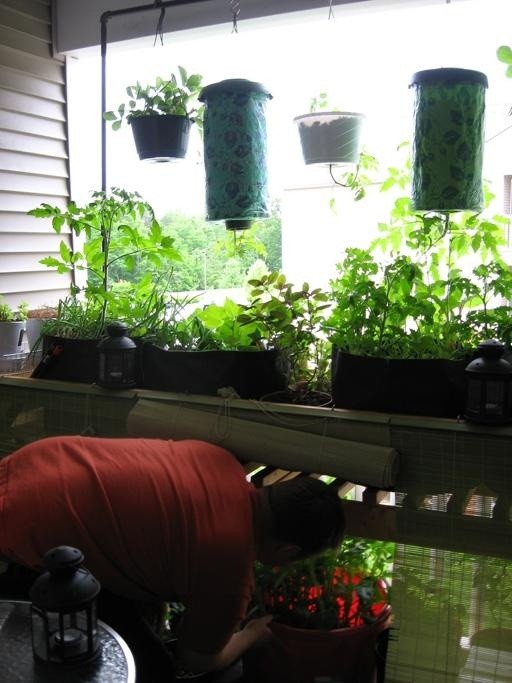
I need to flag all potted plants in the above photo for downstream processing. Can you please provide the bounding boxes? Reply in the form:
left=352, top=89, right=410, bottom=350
left=102, top=63, right=206, bottom=164
left=292, top=91, right=368, bottom=168
left=0, top=182, right=512, bottom=423
left=249, top=534, right=395, bottom=682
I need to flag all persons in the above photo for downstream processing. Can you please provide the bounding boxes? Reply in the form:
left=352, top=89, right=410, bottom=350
left=1, top=436, right=347, bottom=680
